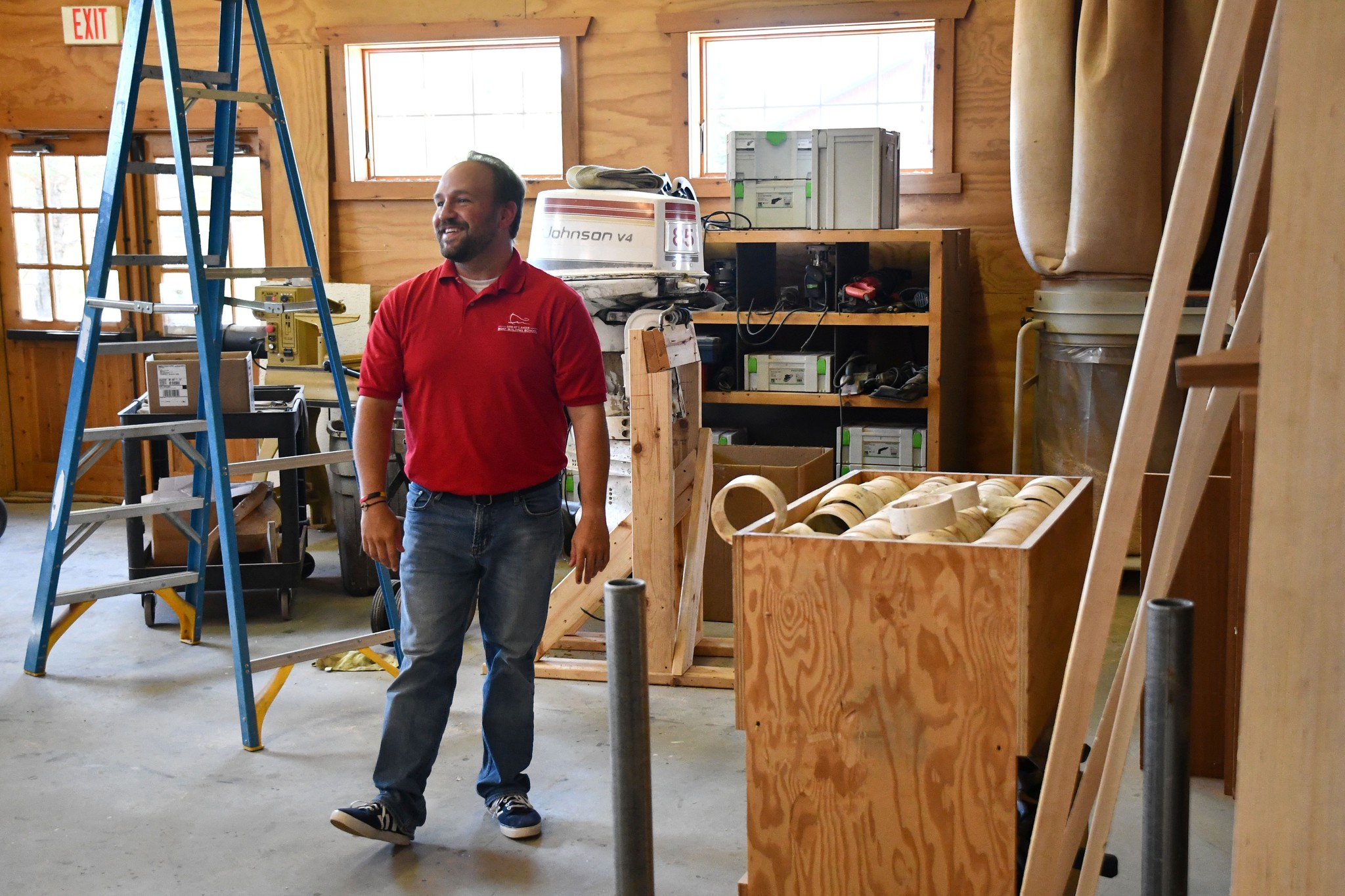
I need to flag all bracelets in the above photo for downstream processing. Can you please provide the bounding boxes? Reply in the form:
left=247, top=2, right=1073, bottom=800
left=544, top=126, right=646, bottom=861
left=359, top=492, right=388, bottom=512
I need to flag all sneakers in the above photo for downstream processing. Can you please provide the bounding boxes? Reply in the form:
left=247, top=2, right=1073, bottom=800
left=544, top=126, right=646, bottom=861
left=330, top=800, right=411, bottom=846
left=487, top=794, right=542, bottom=839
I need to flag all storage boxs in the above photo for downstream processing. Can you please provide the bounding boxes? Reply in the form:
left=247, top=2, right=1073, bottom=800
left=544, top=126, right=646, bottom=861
left=147, top=351, right=254, bottom=412
left=703, top=444, right=833, bottom=623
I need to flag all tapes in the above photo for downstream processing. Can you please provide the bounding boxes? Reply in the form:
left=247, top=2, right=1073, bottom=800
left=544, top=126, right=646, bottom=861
left=803, top=475, right=909, bottom=536
left=779, top=521, right=813, bottom=535
left=888, top=494, right=956, bottom=536
left=840, top=476, right=1074, bottom=546
left=933, top=481, right=981, bottom=511
left=709, top=473, right=787, bottom=546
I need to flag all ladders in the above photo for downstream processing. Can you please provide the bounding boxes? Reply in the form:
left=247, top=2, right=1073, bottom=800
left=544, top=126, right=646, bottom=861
left=21, top=0, right=403, bottom=752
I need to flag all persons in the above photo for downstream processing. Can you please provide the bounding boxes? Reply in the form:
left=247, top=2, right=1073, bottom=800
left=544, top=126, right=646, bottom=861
left=328, top=150, right=610, bottom=847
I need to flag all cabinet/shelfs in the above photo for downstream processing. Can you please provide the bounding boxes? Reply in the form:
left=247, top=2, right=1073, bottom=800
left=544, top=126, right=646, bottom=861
left=668, top=225, right=973, bottom=659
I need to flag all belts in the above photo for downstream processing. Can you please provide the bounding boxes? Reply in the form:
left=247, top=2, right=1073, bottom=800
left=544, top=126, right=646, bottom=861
left=437, top=473, right=559, bottom=505
